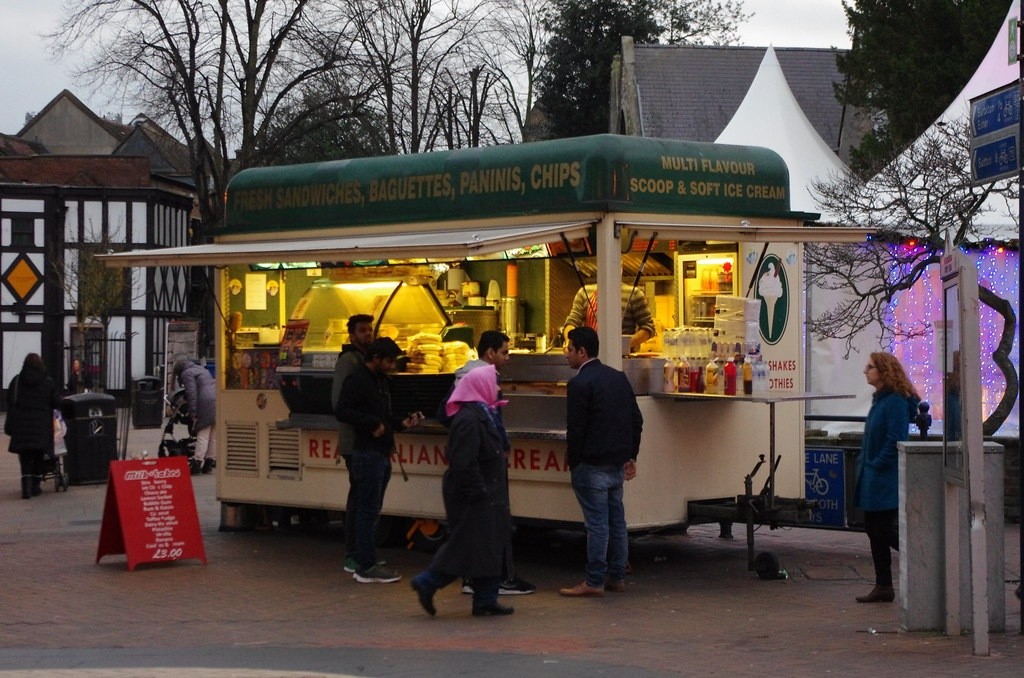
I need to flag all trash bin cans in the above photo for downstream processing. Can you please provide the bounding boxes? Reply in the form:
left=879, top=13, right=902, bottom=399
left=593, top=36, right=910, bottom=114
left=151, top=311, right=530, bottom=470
left=61, top=394, right=118, bottom=486
left=133, top=376, right=164, bottom=430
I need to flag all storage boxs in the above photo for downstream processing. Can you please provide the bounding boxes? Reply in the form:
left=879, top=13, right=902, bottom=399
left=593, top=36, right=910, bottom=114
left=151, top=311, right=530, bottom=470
left=623, top=357, right=666, bottom=394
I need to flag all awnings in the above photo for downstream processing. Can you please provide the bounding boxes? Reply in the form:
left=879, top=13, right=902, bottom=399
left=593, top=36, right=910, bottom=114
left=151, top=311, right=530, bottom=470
left=619, top=221, right=878, bottom=242
left=94, top=220, right=599, bottom=331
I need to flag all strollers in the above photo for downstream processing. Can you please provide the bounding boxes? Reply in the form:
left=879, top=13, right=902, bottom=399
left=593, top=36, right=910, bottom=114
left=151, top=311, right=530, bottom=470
left=157, top=389, right=207, bottom=474
left=41, top=407, right=73, bottom=492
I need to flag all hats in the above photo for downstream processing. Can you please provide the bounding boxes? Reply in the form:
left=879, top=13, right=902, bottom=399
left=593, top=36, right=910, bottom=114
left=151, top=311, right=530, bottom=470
left=369, top=336, right=403, bottom=358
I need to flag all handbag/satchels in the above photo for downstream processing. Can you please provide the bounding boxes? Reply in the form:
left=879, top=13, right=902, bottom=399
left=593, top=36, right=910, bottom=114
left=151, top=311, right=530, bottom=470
left=5, top=375, right=20, bottom=436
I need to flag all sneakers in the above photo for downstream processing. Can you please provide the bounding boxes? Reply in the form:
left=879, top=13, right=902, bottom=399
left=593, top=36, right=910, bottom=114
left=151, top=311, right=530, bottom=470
left=463, top=578, right=476, bottom=594
left=498, top=576, right=536, bottom=595
left=344, top=555, right=385, bottom=572
left=353, top=567, right=401, bottom=583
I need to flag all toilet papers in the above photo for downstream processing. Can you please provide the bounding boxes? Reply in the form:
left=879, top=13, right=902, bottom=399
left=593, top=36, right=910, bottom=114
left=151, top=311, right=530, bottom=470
left=448, top=268, right=466, bottom=290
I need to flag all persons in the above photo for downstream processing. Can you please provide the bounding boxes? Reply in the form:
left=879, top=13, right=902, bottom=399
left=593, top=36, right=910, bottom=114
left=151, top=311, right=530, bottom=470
left=568, top=255, right=655, bottom=350
left=856, top=352, right=919, bottom=601
left=3, top=353, right=56, bottom=500
left=172, top=359, right=216, bottom=473
left=66, top=360, right=94, bottom=394
left=557, top=327, right=644, bottom=596
left=330, top=314, right=403, bottom=584
left=409, top=330, right=537, bottom=616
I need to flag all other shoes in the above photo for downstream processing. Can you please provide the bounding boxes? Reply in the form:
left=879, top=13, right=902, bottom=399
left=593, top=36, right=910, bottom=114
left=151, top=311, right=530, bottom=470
left=855, top=590, right=894, bottom=603
left=560, top=580, right=605, bottom=596
left=472, top=603, right=514, bottom=616
left=411, top=578, right=437, bottom=615
left=606, top=579, right=625, bottom=592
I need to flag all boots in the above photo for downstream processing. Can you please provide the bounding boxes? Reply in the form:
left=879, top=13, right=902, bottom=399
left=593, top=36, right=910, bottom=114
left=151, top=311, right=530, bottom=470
left=32, top=476, right=42, bottom=496
left=21, top=475, right=33, bottom=499
left=191, top=460, right=201, bottom=474
left=202, top=459, right=213, bottom=473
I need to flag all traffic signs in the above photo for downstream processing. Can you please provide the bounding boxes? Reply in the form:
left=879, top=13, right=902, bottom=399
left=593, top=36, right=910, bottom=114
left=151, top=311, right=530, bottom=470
left=968, top=81, right=1022, bottom=138
left=966, top=134, right=1023, bottom=184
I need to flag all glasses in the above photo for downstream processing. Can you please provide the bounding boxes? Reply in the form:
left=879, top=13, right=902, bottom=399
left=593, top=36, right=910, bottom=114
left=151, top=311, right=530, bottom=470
left=868, top=364, right=877, bottom=370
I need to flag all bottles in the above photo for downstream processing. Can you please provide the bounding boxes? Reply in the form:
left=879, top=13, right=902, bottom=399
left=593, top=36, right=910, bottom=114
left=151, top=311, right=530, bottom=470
left=662, top=263, right=770, bottom=396
